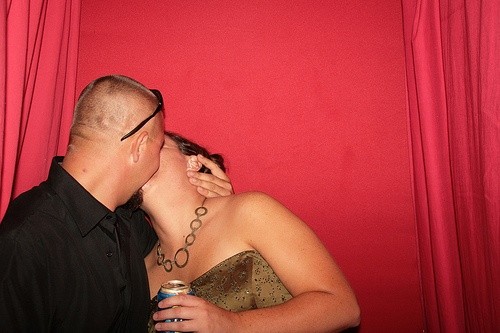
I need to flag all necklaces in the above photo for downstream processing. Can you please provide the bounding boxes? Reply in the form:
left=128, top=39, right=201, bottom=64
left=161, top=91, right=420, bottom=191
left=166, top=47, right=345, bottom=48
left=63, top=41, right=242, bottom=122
left=155, top=197, right=208, bottom=272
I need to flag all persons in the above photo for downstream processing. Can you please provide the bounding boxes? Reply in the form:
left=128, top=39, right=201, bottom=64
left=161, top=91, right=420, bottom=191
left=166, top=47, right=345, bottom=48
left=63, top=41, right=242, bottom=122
left=0, top=74, right=234, bottom=333
left=138, top=131, right=361, bottom=333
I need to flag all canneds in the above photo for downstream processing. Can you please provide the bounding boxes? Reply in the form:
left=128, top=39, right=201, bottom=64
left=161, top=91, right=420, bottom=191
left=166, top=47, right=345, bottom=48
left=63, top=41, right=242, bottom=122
left=157, top=279, right=195, bottom=333
